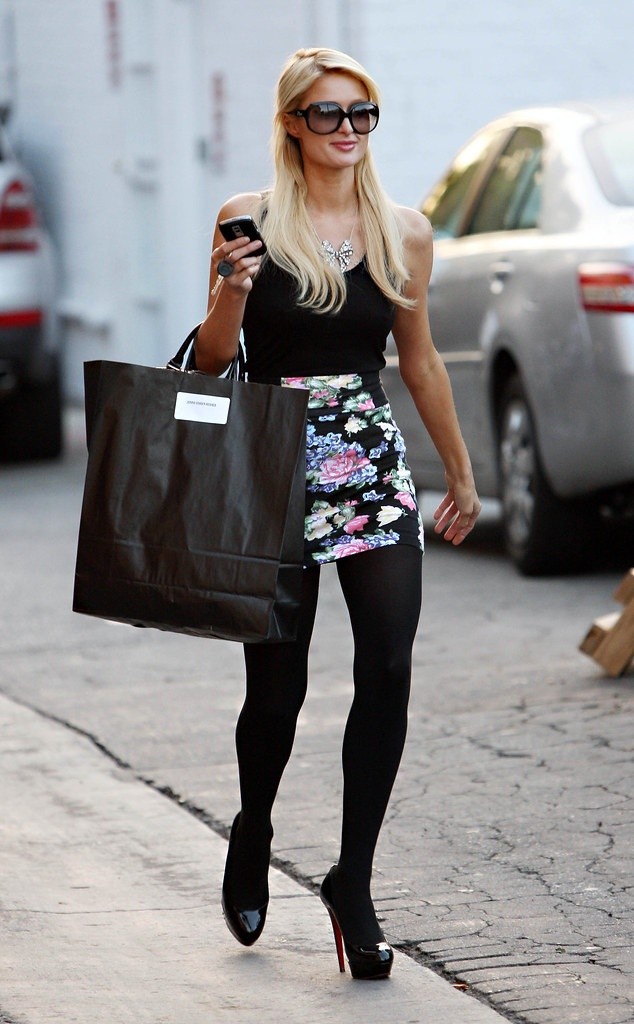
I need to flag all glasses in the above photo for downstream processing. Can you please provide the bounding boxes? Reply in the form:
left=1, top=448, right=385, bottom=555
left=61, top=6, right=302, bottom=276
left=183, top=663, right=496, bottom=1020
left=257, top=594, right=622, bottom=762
left=288, top=100, right=380, bottom=135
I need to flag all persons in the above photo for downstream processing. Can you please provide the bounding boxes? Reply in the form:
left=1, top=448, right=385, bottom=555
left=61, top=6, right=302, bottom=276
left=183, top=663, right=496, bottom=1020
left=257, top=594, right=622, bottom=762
left=189, top=49, right=480, bottom=981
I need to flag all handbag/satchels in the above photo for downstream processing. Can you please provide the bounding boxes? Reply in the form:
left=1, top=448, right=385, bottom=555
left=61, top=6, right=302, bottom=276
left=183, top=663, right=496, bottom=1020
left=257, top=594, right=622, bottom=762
left=73, top=323, right=313, bottom=644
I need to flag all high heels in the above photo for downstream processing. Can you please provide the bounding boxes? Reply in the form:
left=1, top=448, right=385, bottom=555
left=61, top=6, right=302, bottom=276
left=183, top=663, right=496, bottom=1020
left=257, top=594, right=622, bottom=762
left=319, top=865, right=394, bottom=981
left=222, top=810, right=274, bottom=947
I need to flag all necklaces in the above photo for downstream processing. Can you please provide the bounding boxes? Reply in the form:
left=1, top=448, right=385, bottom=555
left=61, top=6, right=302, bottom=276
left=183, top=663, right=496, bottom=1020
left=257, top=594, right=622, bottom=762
left=311, top=204, right=358, bottom=275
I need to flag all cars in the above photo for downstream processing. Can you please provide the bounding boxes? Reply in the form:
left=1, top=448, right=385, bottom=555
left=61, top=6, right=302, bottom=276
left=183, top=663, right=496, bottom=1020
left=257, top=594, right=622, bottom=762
left=0, top=102, right=67, bottom=466
left=382, top=103, right=634, bottom=578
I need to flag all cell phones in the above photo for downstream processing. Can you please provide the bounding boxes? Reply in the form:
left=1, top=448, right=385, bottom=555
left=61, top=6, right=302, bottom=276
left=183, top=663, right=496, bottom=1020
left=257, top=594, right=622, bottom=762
left=218, top=214, right=267, bottom=258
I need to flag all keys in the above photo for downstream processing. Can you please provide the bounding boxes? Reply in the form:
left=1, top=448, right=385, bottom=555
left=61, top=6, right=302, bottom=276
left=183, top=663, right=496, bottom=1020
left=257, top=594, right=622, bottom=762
left=210, top=252, right=235, bottom=296
